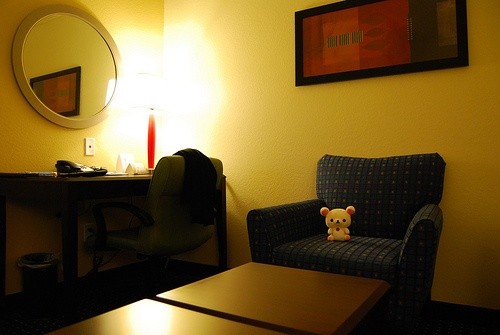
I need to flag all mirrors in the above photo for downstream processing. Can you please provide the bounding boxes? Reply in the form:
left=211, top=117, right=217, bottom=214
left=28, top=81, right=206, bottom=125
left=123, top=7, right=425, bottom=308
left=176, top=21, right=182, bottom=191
left=10, top=5, right=120, bottom=129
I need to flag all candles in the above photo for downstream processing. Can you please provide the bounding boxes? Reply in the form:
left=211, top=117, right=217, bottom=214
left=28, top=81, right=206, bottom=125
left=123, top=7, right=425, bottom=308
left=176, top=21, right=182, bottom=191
left=148, top=107, right=155, bottom=169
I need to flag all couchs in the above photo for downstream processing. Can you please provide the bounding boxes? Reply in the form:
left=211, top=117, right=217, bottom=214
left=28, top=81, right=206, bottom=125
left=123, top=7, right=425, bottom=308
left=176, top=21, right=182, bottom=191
left=247, top=151, right=447, bottom=321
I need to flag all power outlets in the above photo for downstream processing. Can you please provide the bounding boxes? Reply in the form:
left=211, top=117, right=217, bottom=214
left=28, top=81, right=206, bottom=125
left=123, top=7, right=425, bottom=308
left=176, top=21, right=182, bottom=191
left=84, top=223, right=96, bottom=242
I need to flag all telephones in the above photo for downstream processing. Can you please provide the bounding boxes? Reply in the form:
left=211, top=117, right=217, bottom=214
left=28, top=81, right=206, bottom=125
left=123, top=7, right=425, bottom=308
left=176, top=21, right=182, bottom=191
left=56, top=161, right=107, bottom=175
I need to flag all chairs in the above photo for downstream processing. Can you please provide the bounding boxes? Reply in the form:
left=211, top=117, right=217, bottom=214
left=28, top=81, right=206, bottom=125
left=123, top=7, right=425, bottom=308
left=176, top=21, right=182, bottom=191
left=84, top=154, right=223, bottom=301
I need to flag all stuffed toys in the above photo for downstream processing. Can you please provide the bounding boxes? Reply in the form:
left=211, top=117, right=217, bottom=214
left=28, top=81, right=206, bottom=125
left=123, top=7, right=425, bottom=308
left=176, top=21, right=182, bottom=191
left=319, top=206, right=355, bottom=243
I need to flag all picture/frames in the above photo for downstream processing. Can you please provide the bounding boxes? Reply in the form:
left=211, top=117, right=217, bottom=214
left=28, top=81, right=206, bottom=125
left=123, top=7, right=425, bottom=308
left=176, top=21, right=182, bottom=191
left=29, top=67, right=80, bottom=117
left=294, top=0, right=469, bottom=86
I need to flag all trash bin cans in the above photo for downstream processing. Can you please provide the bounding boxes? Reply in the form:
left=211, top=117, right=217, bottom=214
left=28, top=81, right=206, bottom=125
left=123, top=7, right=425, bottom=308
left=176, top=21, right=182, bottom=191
left=20, top=253, right=61, bottom=309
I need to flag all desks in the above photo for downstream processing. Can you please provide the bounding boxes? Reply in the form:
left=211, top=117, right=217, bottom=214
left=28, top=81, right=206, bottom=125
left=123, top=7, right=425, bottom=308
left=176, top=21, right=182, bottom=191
left=1, top=172, right=225, bottom=315
